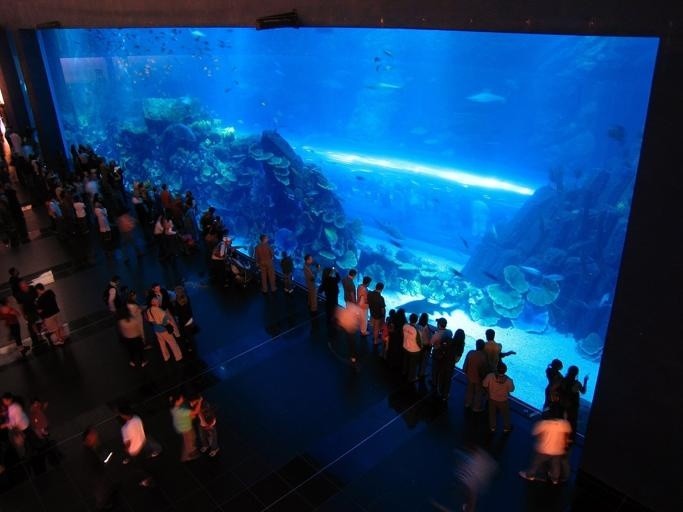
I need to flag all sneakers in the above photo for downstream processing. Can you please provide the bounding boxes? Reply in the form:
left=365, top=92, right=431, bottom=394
left=182, top=446, right=220, bottom=460
left=261, top=287, right=294, bottom=294
left=123, top=450, right=160, bottom=487
left=548, top=472, right=559, bottom=484
left=21, top=338, right=65, bottom=355
left=464, top=400, right=513, bottom=431
left=520, top=472, right=536, bottom=481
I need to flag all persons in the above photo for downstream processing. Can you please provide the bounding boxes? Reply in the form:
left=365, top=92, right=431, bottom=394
left=255, top=235, right=293, bottom=293
left=104, top=277, right=198, bottom=367
left=1, top=267, right=65, bottom=353
left=2, top=129, right=128, bottom=265
left=169, top=393, right=219, bottom=462
left=517, top=358, right=589, bottom=485
left=303, top=255, right=465, bottom=401
left=2, top=394, right=56, bottom=461
left=463, top=329, right=514, bottom=433
left=201, top=209, right=242, bottom=285
left=83, top=409, right=154, bottom=512
left=132, top=183, right=198, bottom=259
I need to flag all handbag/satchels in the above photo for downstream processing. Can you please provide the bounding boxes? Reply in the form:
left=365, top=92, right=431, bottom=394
left=8, top=423, right=26, bottom=448
